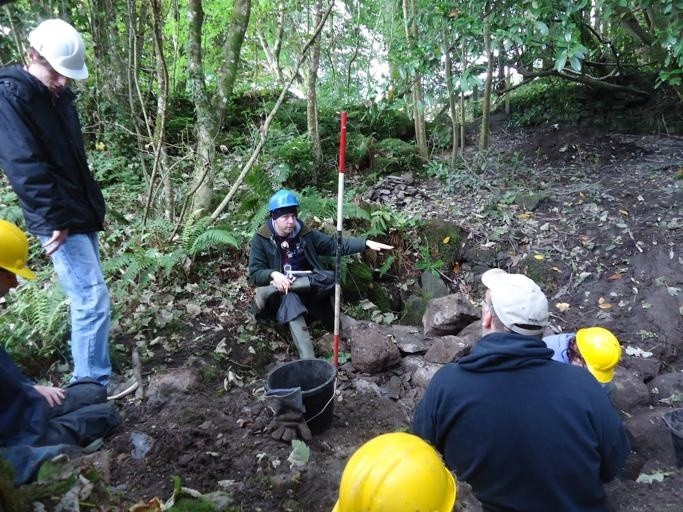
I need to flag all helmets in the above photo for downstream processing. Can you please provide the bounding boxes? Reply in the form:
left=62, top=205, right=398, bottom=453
left=28, top=18, right=90, bottom=79
left=1, top=221, right=37, bottom=281
left=270, top=190, right=301, bottom=211
left=576, top=327, right=621, bottom=384
left=330, top=433, right=455, bottom=512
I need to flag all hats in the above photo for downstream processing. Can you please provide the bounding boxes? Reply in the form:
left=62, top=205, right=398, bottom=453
left=482, top=268, right=549, bottom=335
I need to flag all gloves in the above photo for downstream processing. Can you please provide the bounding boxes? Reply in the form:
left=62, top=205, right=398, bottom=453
left=264, top=388, right=312, bottom=441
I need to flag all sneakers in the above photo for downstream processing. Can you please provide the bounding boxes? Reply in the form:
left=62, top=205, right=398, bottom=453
left=105, top=373, right=138, bottom=401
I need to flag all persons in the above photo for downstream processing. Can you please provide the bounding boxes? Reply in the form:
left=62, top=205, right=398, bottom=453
left=331, top=431, right=483, bottom=512
left=0, top=219, right=124, bottom=489
left=249, top=189, right=395, bottom=359
left=542, top=327, right=621, bottom=404
left=0, top=18, right=138, bottom=400
left=408, top=268, right=631, bottom=512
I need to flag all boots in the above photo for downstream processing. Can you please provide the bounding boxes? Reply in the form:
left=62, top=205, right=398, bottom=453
left=289, top=314, right=316, bottom=361
left=253, top=276, right=311, bottom=309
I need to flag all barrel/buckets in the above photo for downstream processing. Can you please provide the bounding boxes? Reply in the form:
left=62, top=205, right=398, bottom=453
left=263, top=359, right=338, bottom=433
